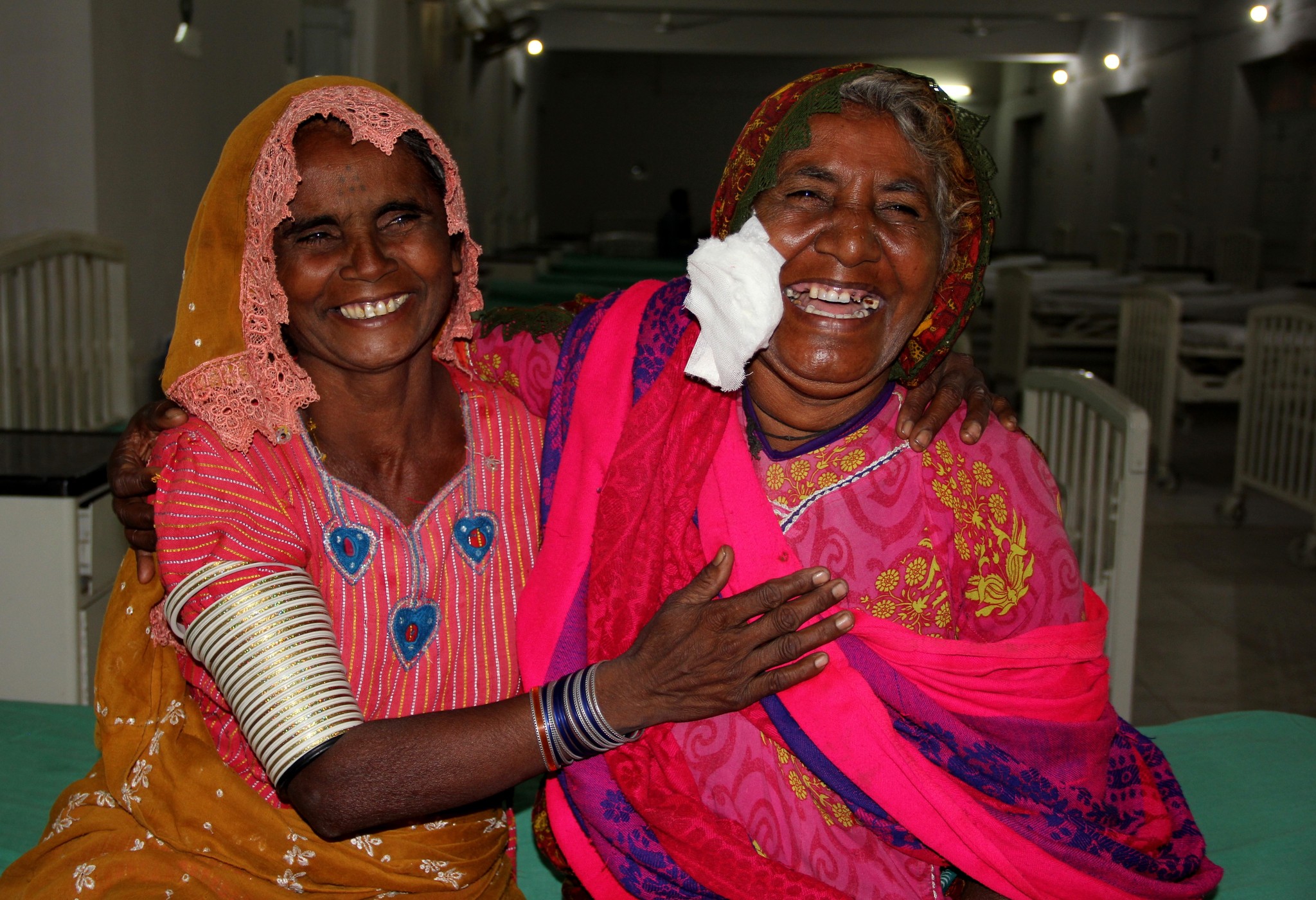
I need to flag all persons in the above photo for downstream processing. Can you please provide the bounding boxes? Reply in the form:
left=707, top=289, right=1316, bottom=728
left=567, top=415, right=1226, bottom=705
left=107, top=68, right=1229, bottom=900
left=3, top=76, right=856, bottom=900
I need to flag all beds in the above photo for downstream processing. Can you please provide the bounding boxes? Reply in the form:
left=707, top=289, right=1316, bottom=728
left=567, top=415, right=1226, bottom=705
left=1, top=692, right=1315, bottom=900
left=466, top=229, right=694, bottom=309
left=963, top=220, right=1316, bottom=555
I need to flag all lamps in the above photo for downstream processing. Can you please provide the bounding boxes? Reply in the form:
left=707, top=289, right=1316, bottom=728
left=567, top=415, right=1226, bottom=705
left=1102, top=50, right=1130, bottom=71
left=928, top=82, right=984, bottom=97
left=1251, top=0, right=1284, bottom=25
left=1052, top=69, right=1076, bottom=85
left=517, top=36, right=544, bottom=58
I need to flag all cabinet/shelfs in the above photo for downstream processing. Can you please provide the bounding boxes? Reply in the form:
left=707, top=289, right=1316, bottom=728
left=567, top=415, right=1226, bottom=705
left=0, top=426, right=130, bottom=708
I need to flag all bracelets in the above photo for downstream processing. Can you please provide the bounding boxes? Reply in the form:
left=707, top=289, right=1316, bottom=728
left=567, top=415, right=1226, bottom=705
left=529, top=660, right=650, bottom=771
